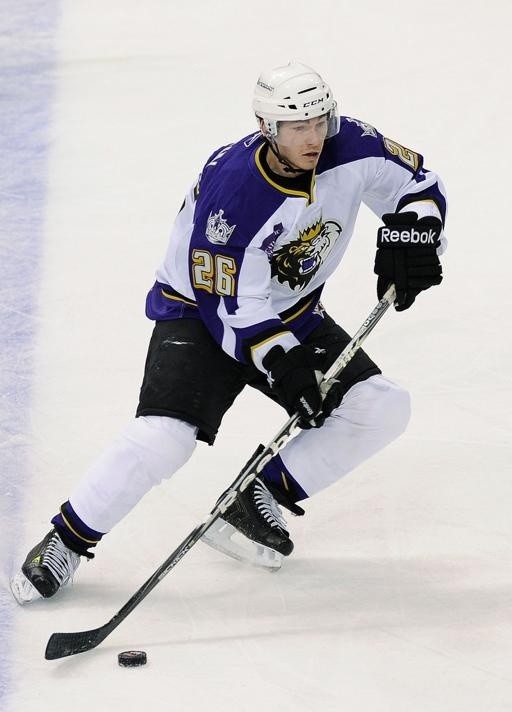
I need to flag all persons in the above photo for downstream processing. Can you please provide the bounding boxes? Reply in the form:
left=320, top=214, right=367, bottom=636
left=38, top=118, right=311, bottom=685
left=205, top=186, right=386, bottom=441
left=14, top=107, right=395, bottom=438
left=19, top=63, right=450, bottom=598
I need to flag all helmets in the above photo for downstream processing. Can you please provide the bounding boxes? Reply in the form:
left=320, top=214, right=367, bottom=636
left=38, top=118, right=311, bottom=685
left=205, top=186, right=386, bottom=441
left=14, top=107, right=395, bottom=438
left=253, top=57, right=341, bottom=147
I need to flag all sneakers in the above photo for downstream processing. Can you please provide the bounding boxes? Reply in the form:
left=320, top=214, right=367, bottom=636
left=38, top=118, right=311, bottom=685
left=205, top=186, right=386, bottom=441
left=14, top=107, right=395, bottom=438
left=23, top=522, right=94, bottom=598
left=215, top=444, right=305, bottom=557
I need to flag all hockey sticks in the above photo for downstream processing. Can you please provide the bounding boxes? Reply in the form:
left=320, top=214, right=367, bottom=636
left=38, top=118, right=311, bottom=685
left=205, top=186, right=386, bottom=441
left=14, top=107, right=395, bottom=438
left=45, top=233, right=448, bottom=660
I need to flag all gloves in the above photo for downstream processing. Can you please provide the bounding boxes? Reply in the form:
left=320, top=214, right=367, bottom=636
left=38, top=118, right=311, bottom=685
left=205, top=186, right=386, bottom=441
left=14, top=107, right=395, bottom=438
left=263, top=343, right=345, bottom=431
left=373, top=210, right=444, bottom=312
